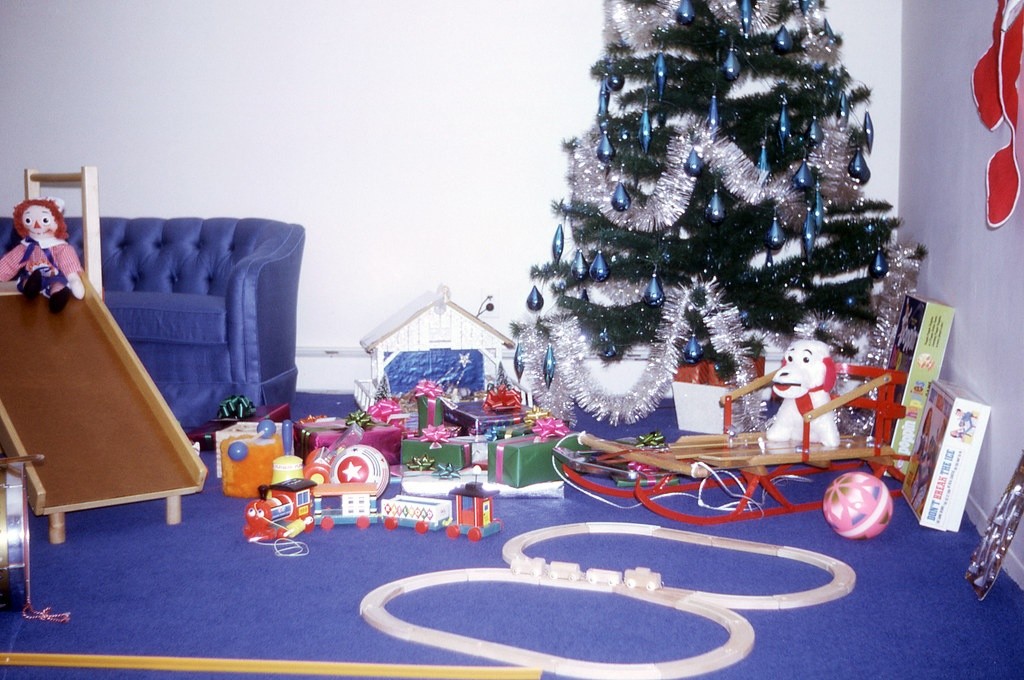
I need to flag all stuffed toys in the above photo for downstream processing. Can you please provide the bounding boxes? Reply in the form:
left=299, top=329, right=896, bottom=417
left=766, top=340, right=841, bottom=448
left=0, top=197, right=86, bottom=313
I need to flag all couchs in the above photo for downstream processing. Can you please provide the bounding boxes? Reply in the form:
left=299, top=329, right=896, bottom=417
left=0, top=217, right=305, bottom=432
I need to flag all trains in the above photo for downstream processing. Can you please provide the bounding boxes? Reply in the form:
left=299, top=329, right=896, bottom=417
left=244, top=474, right=505, bottom=546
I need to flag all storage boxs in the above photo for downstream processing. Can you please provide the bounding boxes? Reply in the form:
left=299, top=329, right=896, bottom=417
left=399, top=425, right=472, bottom=471
left=443, top=384, right=531, bottom=436
left=186, top=394, right=291, bottom=451
left=488, top=415, right=590, bottom=488
left=870, top=292, right=955, bottom=478
left=400, top=462, right=565, bottom=499
left=294, top=408, right=401, bottom=466
left=414, top=379, right=443, bottom=437
left=900, top=376, right=991, bottom=533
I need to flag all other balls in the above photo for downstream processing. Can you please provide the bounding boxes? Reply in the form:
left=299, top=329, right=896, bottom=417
left=822, top=471, right=894, bottom=540
